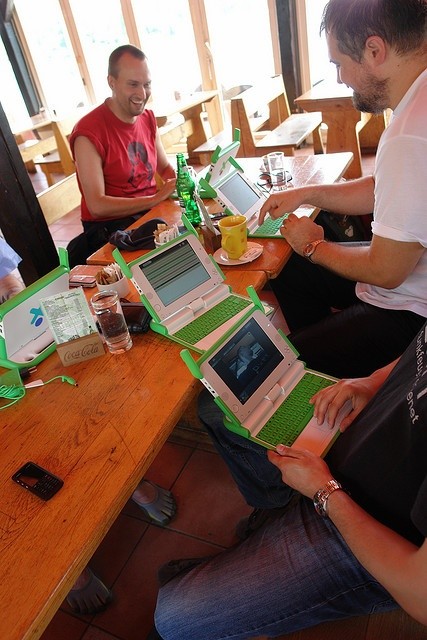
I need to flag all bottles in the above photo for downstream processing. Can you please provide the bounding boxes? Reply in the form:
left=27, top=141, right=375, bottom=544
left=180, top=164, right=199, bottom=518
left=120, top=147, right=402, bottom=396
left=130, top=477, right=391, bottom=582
left=175, top=153, right=201, bottom=229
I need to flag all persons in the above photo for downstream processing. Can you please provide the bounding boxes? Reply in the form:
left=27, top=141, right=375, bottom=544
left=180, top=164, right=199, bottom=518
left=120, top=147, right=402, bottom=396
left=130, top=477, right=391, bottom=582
left=155, top=301, right=426, bottom=635
left=1, top=228, right=178, bottom=617
left=67, top=46, right=177, bottom=248
left=260, top=1, right=426, bottom=371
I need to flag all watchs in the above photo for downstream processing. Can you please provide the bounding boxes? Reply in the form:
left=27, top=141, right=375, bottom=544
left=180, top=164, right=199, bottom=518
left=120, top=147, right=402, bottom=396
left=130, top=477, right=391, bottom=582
left=311, top=480, right=341, bottom=519
left=301, top=237, right=325, bottom=264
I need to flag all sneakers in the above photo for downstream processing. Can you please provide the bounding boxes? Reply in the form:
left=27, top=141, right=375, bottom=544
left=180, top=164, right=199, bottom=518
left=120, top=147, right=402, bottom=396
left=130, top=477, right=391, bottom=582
left=237, top=503, right=281, bottom=537
left=158, top=555, right=213, bottom=584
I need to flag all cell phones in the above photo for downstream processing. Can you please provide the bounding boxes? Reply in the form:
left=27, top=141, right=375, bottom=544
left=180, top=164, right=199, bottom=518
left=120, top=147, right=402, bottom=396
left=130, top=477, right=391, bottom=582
left=70, top=274, right=98, bottom=289
left=12, top=461, right=68, bottom=501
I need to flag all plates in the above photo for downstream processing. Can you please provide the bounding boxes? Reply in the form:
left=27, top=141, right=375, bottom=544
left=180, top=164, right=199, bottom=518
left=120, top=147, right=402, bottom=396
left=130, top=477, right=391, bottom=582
left=212, top=241, right=263, bottom=266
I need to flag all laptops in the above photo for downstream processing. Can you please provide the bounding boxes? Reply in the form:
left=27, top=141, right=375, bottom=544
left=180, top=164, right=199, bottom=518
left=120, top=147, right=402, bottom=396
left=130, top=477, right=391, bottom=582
left=1, top=247, right=71, bottom=371
left=199, top=156, right=316, bottom=240
left=111, top=213, right=275, bottom=356
left=180, top=285, right=353, bottom=460
left=171, top=128, right=239, bottom=200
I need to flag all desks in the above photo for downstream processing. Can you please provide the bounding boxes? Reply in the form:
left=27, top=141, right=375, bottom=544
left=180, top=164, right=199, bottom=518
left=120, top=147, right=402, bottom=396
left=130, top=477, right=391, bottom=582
left=85, top=152, right=354, bottom=279
left=0, top=263, right=267, bottom=640
left=151, top=91, right=220, bottom=155
left=294, top=73, right=386, bottom=178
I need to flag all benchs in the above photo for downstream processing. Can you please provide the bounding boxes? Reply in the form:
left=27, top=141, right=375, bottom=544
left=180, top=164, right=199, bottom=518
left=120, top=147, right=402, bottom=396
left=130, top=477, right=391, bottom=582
left=239, top=72, right=323, bottom=156
left=193, top=74, right=278, bottom=164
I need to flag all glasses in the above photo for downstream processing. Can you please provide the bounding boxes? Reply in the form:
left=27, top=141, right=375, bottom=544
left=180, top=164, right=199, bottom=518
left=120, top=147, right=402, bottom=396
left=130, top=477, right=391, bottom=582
left=256, top=168, right=292, bottom=197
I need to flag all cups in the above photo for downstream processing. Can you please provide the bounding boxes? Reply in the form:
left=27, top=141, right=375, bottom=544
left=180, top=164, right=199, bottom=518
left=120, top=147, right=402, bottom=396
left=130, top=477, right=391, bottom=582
left=153, top=232, right=181, bottom=249
left=260, top=155, right=275, bottom=172
left=267, top=151, right=287, bottom=193
left=91, top=290, right=134, bottom=355
left=95, top=273, right=130, bottom=298
left=217, top=214, right=248, bottom=261
left=174, top=165, right=197, bottom=179
